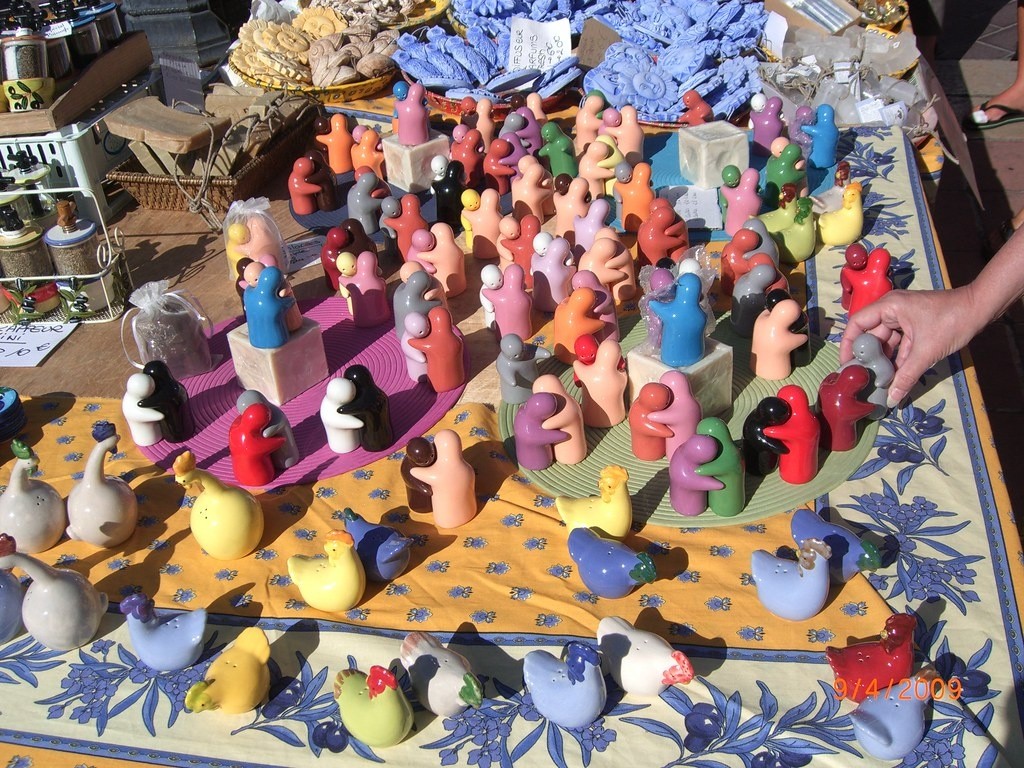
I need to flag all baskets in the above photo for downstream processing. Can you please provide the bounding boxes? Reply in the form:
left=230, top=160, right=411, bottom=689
left=105, top=109, right=317, bottom=210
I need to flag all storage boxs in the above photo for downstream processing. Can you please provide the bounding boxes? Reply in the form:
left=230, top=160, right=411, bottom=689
left=0, top=28, right=170, bottom=226
left=764, top=0, right=862, bottom=37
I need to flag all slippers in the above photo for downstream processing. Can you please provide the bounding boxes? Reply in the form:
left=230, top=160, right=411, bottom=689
left=964, top=100, right=1024, bottom=129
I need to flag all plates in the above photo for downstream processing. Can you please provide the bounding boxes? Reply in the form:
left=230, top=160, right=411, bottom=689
left=581, top=55, right=752, bottom=128
left=401, top=38, right=574, bottom=121
left=229, top=46, right=397, bottom=103
left=298, top=2, right=455, bottom=29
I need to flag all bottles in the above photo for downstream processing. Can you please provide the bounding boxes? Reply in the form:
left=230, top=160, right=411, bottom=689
left=1, top=2, right=123, bottom=103
left=0, top=163, right=115, bottom=314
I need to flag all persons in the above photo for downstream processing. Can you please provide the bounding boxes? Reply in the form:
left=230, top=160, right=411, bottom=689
left=961, top=0, right=1024, bottom=129
left=838, top=208, right=1024, bottom=408
